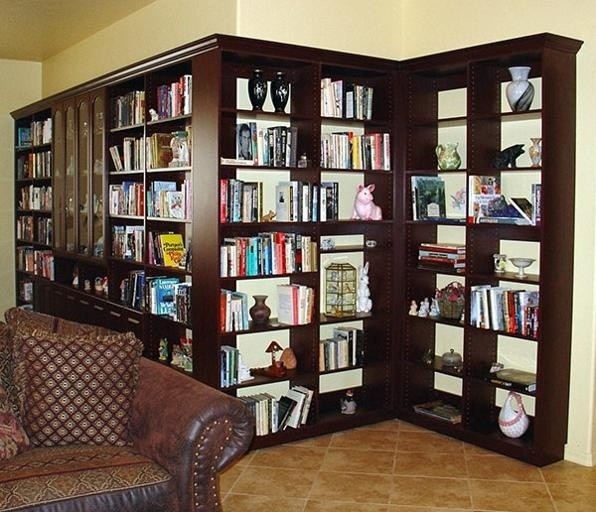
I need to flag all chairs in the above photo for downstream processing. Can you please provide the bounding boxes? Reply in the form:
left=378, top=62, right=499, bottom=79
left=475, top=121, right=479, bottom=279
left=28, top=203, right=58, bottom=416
left=1, top=316, right=258, bottom=512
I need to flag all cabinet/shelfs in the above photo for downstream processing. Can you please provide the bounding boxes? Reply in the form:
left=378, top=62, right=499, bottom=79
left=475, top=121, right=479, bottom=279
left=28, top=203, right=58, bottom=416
left=9, top=32, right=586, bottom=468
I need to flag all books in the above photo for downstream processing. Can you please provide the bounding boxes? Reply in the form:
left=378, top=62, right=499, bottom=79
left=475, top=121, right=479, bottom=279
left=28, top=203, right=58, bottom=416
left=487, top=369, right=536, bottom=393
left=220, top=345, right=314, bottom=437
left=276, top=283, right=314, bottom=326
left=220, top=288, right=250, bottom=334
left=15, top=118, right=55, bottom=282
left=410, top=174, right=541, bottom=341
left=219, top=122, right=298, bottom=167
left=274, top=181, right=339, bottom=222
left=320, top=129, right=391, bottom=171
left=321, top=76, right=374, bottom=120
left=219, top=178, right=263, bottom=223
left=319, top=326, right=364, bottom=372
left=219, top=231, right=318, bottom=278
left=109, top=72, right=192, bottom=325
